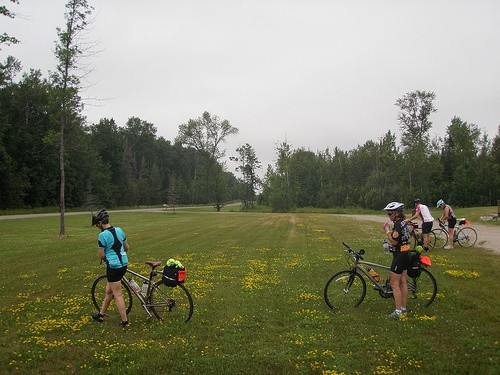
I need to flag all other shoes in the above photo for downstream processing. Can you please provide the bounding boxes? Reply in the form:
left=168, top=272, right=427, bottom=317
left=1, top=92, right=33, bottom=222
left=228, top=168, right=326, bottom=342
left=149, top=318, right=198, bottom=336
left=424, top=248, right=429, bottom=252
left=389, top=309, right=407, bottom=318
left=444, top=245, right=453, bottom=250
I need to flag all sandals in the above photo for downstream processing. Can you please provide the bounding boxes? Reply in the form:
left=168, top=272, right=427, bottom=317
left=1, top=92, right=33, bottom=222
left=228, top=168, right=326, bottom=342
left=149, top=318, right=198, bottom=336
left=119, top=321, right=129, bottom=328
left=92, top=313, right=104, bottom=323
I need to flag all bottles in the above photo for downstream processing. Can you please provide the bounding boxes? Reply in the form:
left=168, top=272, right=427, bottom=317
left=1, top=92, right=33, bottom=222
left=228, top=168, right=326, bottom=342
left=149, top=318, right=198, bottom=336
left=367, top=267, right=382, bottom=283
left=385, top=224, right=389, bottom=233
left=141, top=280, right=149, bottom=297
left=382, top=239, right=390, bottom=255
left=128, top=278, right=141, bottom=293
left=415, top=230, right=418, bottom=238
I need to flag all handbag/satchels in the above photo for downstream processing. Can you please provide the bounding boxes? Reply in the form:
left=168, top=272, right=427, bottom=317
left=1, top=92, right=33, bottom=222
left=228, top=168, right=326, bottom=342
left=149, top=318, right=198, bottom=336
left=162, top=265, right=187, bottom=288
left=407, top=250, right=420, bottom=278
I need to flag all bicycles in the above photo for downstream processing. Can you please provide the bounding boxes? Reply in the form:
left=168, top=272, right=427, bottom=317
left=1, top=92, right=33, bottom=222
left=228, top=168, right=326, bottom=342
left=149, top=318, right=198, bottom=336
left=324, top=243, right=438, bottom=311
left=409, top=221, right=436, bottom=251
left=429, top=217, right=477, bottom=248
left=92, top=259, right=193, bottom=324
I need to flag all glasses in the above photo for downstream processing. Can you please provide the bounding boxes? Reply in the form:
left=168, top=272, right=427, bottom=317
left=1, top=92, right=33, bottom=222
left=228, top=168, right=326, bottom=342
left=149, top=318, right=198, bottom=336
left=388, top=212, right=395, bottom=216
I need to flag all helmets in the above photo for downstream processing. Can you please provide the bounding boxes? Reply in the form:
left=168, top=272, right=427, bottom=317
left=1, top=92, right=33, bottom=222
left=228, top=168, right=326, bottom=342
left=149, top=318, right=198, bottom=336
left=437, top=199, right=443, bottom=209
left=92, top=209, right=109, bottom=226
left=384, top=202, right=404, bottom=211
left=413, top=199, right=421, bottom=205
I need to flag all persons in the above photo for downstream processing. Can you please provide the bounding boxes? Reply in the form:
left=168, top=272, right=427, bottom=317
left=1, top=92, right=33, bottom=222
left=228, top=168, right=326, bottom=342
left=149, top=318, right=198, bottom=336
left=406, top=199, right=434, bottom=252
left=92, top=208, right=130, bottom=327
left=436, top=199, right=457, bottom=249
left=382, top=202, right=411, bottom=319
